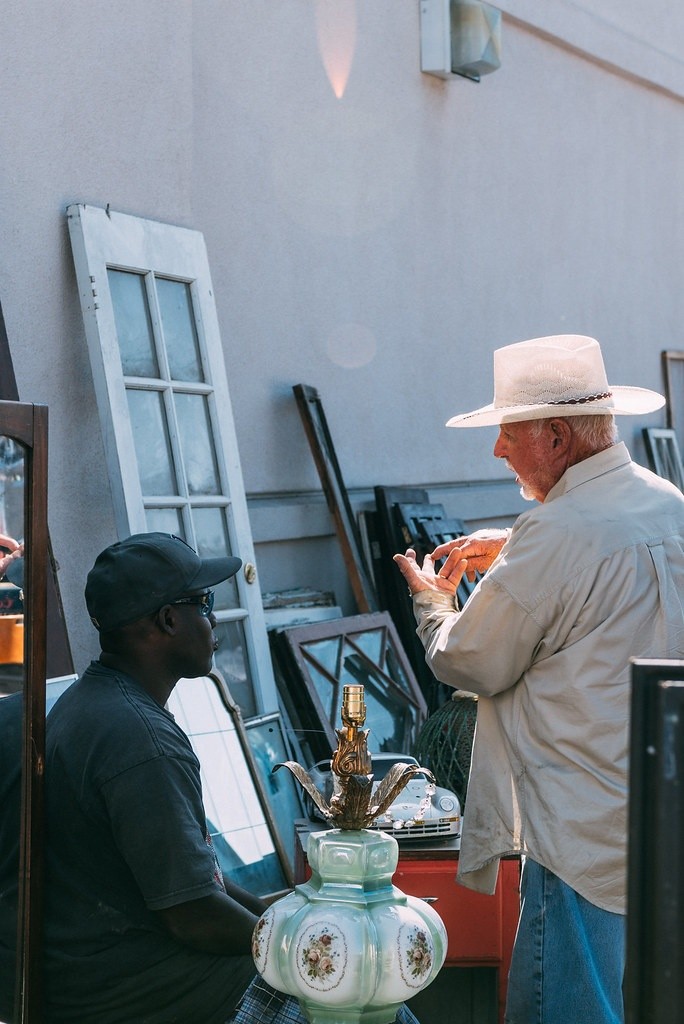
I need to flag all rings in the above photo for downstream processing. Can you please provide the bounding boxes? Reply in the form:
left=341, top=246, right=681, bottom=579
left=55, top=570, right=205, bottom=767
left=438, top=573, right=447, bottom=579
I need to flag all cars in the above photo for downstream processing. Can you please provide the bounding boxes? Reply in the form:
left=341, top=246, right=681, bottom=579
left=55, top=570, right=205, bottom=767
left=301, top=751, right=462, bottom=845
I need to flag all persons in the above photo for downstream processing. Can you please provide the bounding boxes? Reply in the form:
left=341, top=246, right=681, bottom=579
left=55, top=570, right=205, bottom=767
left=43, top=533, right=420, bottom=1024
left=393, top=334, right=684, bottom=1024
left=0, top=535, right=24, bottom=615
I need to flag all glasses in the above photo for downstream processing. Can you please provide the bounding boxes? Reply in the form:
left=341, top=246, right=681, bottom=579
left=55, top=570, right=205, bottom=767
left=169, top=591, right=215, bottom=617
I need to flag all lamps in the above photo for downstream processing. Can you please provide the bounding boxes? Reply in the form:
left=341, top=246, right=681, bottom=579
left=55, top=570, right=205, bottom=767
left=419, top=0, right=501, bottom=83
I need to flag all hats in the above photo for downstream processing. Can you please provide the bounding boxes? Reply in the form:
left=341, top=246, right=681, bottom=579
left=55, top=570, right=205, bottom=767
left=4, top=556, right=25, bottom=590
left=445, top=334, right=666, bottom=428
left=85, top=532, right=242, bottom=630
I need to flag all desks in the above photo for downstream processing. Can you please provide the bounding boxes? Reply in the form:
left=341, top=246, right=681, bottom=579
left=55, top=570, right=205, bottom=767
left=305, top=818, right=522, bottom=1024
